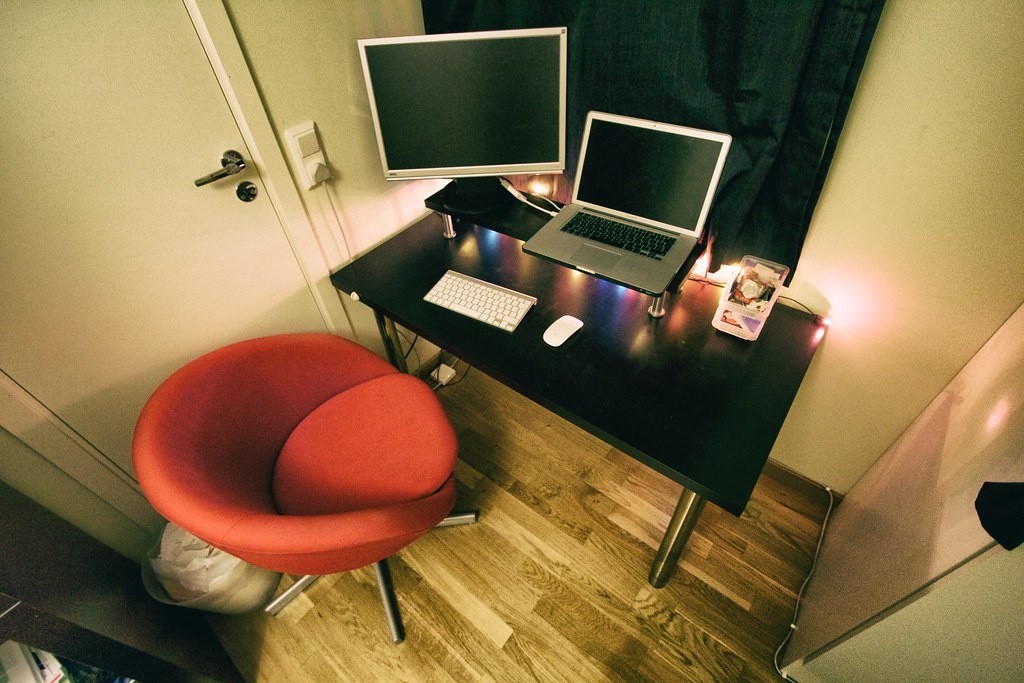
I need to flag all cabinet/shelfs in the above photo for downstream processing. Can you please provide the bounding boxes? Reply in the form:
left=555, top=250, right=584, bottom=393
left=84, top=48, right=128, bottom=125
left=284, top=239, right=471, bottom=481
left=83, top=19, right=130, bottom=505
left=780, top=302, right=1024, bottom=683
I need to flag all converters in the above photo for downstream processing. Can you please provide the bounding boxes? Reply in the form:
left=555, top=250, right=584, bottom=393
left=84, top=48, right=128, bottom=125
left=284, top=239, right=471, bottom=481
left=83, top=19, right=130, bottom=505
left=431, top=364, right=456, bottom=385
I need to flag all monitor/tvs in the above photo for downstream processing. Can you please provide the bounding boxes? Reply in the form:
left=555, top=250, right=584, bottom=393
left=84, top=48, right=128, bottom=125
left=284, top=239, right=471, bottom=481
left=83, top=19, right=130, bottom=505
left=357, top=26, right=567, bottom=213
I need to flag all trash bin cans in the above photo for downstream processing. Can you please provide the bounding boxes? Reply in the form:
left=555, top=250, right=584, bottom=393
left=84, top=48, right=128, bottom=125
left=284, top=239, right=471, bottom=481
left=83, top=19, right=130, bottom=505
left=138, top=523, right=283, bottom=622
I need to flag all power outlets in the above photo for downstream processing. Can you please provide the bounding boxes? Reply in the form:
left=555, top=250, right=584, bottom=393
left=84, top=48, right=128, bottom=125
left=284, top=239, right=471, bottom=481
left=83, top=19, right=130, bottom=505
left=283, top=119, right=335, bottom=192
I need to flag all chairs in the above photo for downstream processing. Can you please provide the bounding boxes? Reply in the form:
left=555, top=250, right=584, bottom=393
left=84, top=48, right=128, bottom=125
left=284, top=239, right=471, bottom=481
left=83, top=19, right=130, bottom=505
left=133, top=332, right=478, bottom=644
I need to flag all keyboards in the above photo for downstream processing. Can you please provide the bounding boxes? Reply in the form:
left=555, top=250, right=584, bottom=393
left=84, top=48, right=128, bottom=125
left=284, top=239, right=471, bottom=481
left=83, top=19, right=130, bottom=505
left=423, top=269, right=538, bottom=332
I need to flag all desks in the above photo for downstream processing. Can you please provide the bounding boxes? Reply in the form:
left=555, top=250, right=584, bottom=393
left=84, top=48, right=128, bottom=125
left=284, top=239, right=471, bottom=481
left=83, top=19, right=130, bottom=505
left=329, top=176, right=828, bottom=589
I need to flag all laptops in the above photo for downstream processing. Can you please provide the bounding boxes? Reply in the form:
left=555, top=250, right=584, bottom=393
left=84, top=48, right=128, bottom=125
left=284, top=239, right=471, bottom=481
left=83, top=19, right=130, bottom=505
left=522, top=111, right=732, bottom=296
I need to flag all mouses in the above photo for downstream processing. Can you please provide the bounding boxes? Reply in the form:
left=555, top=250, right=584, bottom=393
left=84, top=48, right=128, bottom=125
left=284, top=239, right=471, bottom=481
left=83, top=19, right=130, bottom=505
left=543, top=315, right=584, bottom=347
